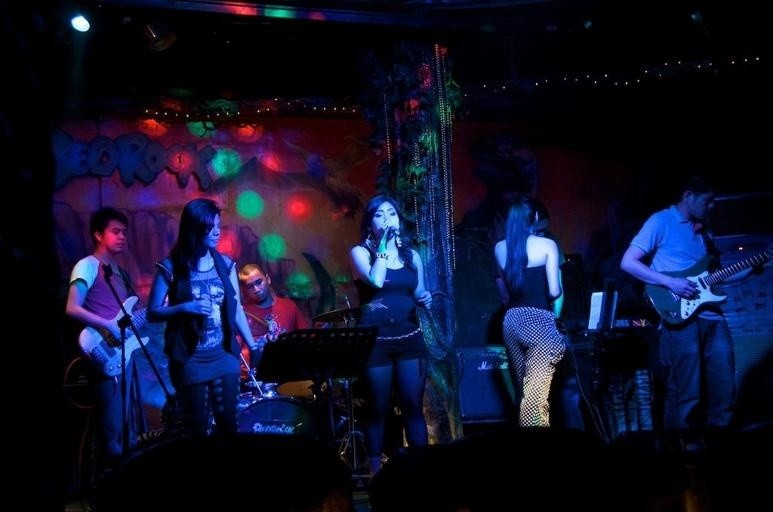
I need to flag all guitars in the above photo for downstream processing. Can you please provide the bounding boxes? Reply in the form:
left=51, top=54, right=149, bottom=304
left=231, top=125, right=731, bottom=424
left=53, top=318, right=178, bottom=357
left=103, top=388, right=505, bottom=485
left=79, top=296, right=168, bottom=377
left=644, top=247, right=773, bottom=326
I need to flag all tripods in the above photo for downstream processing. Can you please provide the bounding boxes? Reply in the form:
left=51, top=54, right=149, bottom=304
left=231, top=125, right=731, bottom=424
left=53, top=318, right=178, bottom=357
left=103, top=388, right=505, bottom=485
left=340, top=380, right=367, bottom=470
left=117, top=328, right=168, bottom=456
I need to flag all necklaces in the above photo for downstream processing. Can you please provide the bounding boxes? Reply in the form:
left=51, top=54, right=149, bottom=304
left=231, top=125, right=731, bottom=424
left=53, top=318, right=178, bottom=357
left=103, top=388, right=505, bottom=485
left=197, top=255, right=212, bottom=294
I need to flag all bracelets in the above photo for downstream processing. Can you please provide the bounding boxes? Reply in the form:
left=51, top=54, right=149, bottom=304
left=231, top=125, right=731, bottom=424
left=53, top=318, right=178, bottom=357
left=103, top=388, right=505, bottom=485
left=375, top=251, right=389, bottom=260
left=250, top=343, right=257, bottom=349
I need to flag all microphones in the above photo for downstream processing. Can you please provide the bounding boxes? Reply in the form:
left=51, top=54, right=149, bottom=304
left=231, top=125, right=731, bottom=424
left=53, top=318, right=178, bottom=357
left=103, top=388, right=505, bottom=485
left=201, top=293, right=210, bottom=342
left=388, top=226, right=400, bottom=236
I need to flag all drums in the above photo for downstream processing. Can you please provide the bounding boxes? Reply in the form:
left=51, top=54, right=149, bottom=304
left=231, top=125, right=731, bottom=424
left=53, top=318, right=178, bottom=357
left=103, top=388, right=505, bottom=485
left=235, top=388, right=306, bottom=436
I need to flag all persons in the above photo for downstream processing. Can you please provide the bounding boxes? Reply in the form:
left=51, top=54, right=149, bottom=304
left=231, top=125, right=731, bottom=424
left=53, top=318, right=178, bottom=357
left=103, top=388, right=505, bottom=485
left=145, top=198, right=258, bottom=429
left=494, top=200, right=565, bottom=426
left=621, top=177, right=738, bottom=436
left=351, top=196, right=431, bottom=476
left=233, top=263, right=309, bottom=389
left=66, top=207, right=134, bottom=461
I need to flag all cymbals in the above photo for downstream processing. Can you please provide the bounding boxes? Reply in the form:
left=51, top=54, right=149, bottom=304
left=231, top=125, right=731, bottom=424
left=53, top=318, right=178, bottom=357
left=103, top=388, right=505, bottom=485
left=312, top=306, right=361, bottom=323
left=275, top=379, right=315, bottom=399
